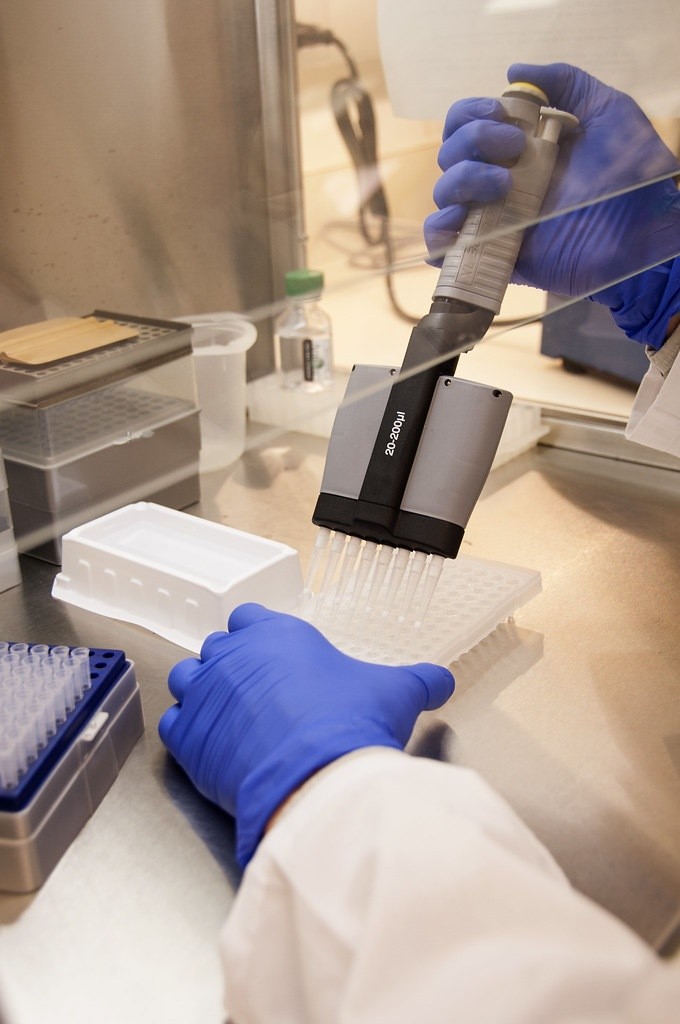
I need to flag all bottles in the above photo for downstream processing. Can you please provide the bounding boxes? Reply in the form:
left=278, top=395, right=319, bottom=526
left=277, top=270, right=333, bottom=400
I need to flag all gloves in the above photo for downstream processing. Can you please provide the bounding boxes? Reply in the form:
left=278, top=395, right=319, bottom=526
left=422, top=63, right=680, bottom=354
left=158, top=601, right=455, bottom=871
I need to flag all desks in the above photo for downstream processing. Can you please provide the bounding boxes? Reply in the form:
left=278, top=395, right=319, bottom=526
left=0, top=263, right=679, bottom=1024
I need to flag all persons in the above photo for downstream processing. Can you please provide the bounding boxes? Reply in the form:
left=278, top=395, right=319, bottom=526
left=157, top=60, right=680, bottom=1024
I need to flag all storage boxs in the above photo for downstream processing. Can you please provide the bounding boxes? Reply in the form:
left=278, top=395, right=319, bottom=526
left=0, top=310, right=201, bottom=566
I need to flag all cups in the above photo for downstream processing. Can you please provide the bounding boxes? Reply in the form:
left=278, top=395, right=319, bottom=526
left=171, top=318, right=257, bottom=473
